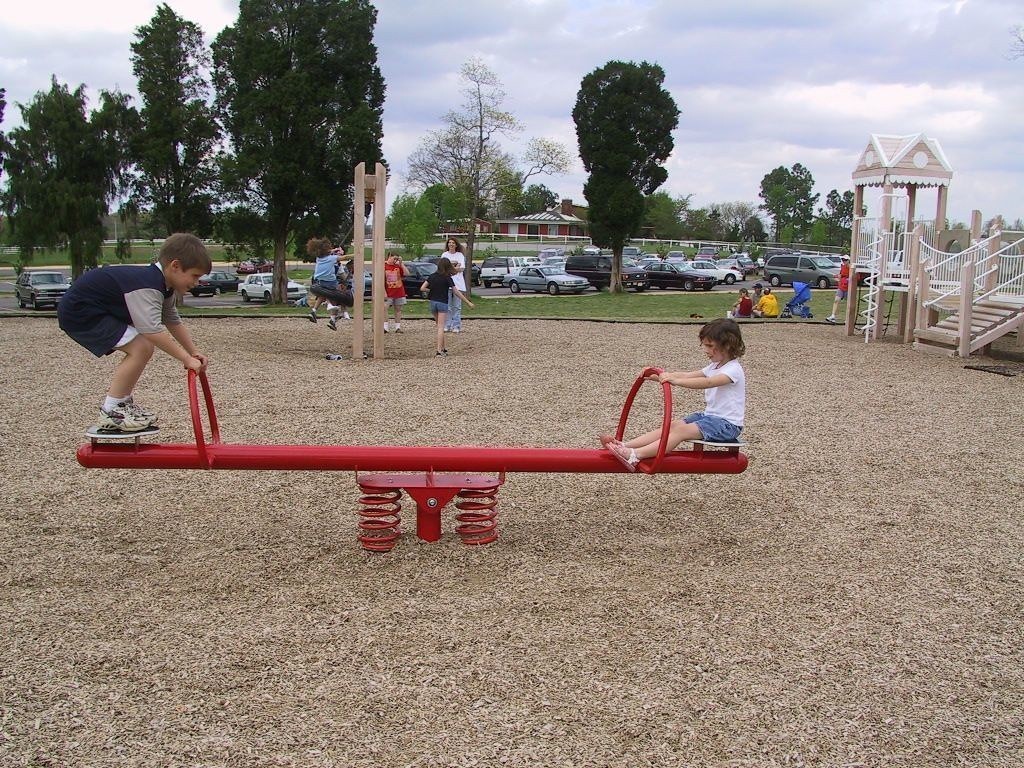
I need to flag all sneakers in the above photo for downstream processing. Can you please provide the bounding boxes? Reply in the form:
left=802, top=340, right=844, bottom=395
left=97, top=406, right=150, bottom=432
left=825, top=317, right=835, bottom=322
left=309, top=312, right=318, bottom=323
left=327, top=354, right=341, bottom=360
left=125, top=397, right=158, bottom=423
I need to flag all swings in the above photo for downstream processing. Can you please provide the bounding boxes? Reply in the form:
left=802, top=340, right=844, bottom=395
left=310, top=215, right=373, bottom=307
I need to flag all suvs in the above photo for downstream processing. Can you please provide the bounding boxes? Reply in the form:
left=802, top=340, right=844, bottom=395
left=762, top=254, right=842, bottom=289
left=14, top=271, right=72, bottom=310
left=565, top=254, right=648, bottom=292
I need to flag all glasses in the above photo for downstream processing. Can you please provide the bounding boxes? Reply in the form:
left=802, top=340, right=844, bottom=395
left=841, top=259, right=848, bottom=261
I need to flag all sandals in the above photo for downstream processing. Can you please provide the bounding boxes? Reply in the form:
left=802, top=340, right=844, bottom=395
left=606, top=442, right=640, bottom=472
left=600, top=433, right=625, bottom=447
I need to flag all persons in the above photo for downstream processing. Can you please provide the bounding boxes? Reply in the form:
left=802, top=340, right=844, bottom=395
left=57, top=233, right=213, bottom=432
left=600, top=321, right=746, bottom=472
left=384, top=252, right=409, bottom=334
left=307, top=237, right=355, bottom=331
left=826, top=254, right=860, bottom=322
left=441, top=237, right=466, bottom=333
left=731, top=283, right=779, bottom=318
left=419, top=258, right=474, bottom=355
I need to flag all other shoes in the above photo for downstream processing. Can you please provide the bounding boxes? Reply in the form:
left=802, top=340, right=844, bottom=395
left=453, top=329, right=459, bottom=333
left=437, top=351, right=448, bottom=357
left=395, top=328, right=402, bottom=333
left=327, top=319, right=337, bottom=330
left=444, top=329, right=449, bottom=331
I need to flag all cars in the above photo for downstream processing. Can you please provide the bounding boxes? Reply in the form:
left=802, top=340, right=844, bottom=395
left=189, top=271, right=245, bottom=297
left=501, top=265, right=591, bottom=296
left=235, top=257, right=274, bottom=274
left=403, top=261, right=438, bottom=299
left=347, top=270, right=373, bottom=297
left=237, top=272, right=308, bottom=304
left=421, top=244, right=933, bottom=288
left=642, top=263, right=718, bottom=292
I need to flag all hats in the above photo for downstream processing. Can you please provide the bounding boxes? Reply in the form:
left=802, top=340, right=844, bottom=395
left=752, top=284, right=762, bottom=288
left=841, top=255, right=850, bottom=259
